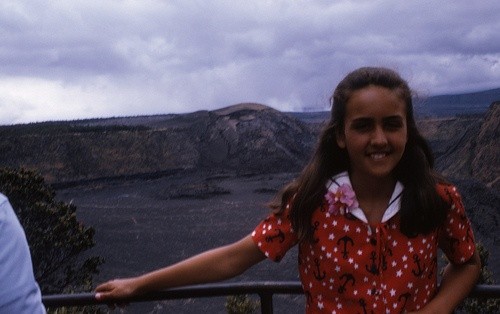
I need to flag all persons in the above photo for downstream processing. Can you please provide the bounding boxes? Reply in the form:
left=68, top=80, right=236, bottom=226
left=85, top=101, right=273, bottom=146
left=0, top=191, right=46, bottom=314
left=96, top=66, right=482, bottom=314
